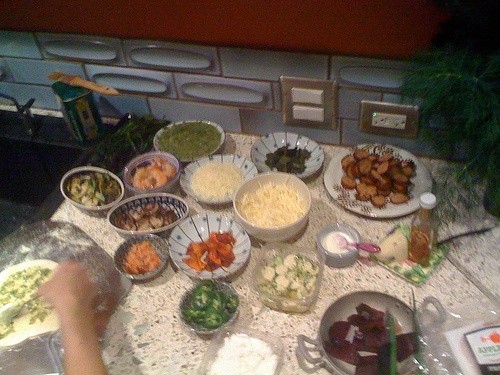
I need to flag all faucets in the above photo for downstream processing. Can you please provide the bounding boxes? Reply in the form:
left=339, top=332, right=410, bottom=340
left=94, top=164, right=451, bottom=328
left=0, top=91, right=20, bottom=110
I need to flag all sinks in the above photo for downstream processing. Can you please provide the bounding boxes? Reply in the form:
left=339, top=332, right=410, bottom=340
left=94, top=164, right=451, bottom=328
left=0, top=133, right=95, bottom=221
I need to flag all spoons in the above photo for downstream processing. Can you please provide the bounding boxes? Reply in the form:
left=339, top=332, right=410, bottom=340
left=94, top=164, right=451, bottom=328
left=334, top=235, right=381, bottom=254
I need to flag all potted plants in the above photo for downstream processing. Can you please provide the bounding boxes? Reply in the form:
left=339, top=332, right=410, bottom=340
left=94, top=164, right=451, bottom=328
left=399, top=0, right=500, bottom=236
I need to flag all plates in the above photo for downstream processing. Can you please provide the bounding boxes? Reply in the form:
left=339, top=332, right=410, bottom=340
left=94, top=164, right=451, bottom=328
left=324, top=143, right=432, bottom=218
left=250, top=131, right=324, bottom=178
left=169, top=214, right=252, bottom=280
left=179, top=280, right=239, bottom=334
left=153, top=120, right=225, bottom=161
left=180, top=154, right=258, bottom=203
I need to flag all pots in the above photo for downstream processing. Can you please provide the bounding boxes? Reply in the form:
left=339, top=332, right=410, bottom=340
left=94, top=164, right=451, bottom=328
left=297, top=291, right=446, bottom=375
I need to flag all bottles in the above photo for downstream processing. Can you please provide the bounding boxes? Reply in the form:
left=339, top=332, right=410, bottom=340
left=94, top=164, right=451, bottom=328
left=408, top=192, right=440, bottom=262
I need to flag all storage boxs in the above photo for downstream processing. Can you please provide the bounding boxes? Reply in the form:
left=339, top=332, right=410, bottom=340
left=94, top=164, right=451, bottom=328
left=52, top=81, right=106, bottom=145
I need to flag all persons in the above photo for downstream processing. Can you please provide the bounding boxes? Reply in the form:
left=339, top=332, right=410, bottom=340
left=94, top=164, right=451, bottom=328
left=36, top=262, right=110, bottom=375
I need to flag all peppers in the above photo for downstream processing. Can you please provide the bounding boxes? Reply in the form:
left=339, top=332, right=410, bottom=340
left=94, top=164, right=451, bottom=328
left=182, top=278, right=239, bottom=330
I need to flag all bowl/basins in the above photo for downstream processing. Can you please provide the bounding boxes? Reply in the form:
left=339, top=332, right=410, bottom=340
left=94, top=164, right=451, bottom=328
left=113, top=234, right=168, bottom=280
left=107, top=193, right=189, bottom=239
left=233, top=173, right=311, bottom=241
left=196, top=325, right=284, bottom=375
left=316, top=223, right=360, bottom=267
left=60, top=166, right=124, bottom=210
left=123, top=152, right=180, bottom=193
left=249, top=242, right=322, bottom=312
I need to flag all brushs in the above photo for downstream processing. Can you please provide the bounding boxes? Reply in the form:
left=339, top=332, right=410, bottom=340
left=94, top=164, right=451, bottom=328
left=48, top=70, right=122, bottom=96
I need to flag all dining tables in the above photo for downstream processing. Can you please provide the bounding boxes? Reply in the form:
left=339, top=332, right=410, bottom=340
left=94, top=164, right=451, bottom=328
left=48, top=133, right=500, bottom=375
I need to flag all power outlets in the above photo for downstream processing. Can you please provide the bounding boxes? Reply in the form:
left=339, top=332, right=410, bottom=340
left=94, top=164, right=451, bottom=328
left=359, top=101, right=420, bottom=139
